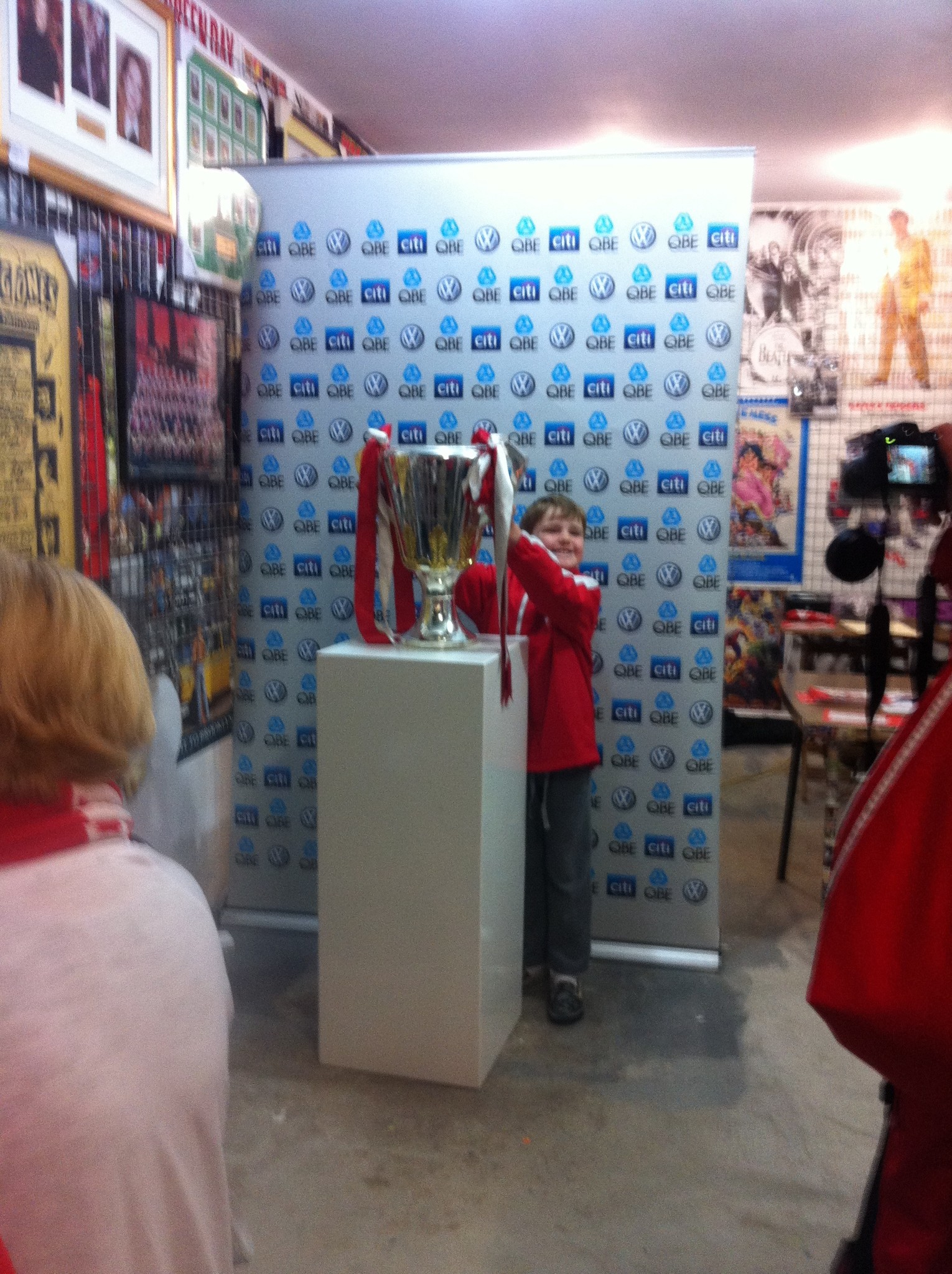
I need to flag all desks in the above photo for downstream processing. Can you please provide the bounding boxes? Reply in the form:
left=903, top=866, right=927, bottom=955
left=842, top=619, right=921, bottom=671
left=777, top=673, right=934, bottom=880
left=319, top=634, right=529, bottom=1089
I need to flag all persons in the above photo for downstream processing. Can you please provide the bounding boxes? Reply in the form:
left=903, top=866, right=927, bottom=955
left=0, top=544, right=235, bottom=1274
left=863, top=207, right=933, bottom=389
left=118, top=46, right=151, bottom=151
left=804, top=662, right=952, bottom=1274
left=128, top=362, right=224, bottom=462
left=71, top=0, right=110, bottom=107
left=20, top=0, right=60, bottom=100
left=452, top=492, right=602, bottom=1025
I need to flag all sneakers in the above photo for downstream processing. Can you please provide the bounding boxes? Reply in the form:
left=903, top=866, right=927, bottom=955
left=550, top=975, right=585, bottom=1023
left=522, top=966, right=545, bottom=995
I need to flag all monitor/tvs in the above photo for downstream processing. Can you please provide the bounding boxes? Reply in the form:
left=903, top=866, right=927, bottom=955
left=120, top=288, right=230, bottom=484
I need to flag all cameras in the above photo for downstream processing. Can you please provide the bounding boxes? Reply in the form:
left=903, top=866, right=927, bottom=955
left=840, top=421, right=950, bottom=497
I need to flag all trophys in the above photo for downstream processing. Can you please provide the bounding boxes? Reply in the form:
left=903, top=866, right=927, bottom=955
left=366, top=429, right=529, bottom=649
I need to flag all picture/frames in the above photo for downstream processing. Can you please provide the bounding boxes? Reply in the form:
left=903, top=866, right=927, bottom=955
left=123, top=288, right=228, bottom=482
